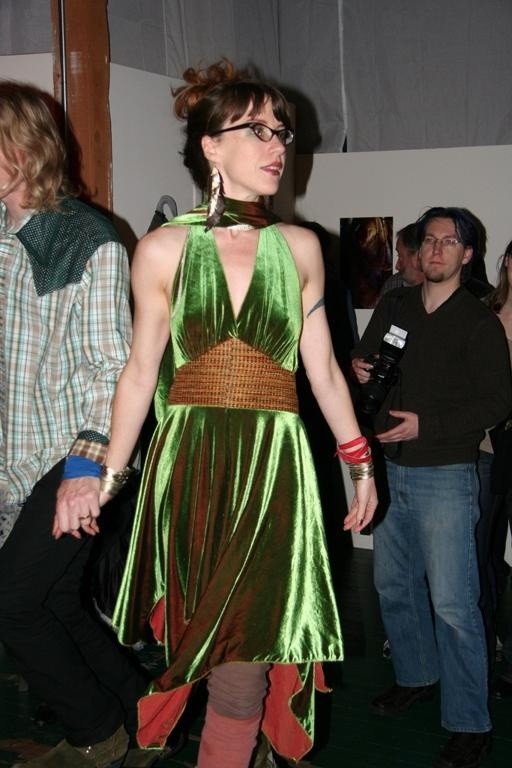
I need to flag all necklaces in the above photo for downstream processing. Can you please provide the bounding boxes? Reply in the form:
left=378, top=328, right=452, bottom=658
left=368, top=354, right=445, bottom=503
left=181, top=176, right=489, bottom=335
left=226, top=221, right=254, bottom=232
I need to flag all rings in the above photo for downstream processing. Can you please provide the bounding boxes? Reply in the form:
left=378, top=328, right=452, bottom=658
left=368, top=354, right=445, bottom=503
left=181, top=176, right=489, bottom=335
left=80, top=513, right=91, bottom=521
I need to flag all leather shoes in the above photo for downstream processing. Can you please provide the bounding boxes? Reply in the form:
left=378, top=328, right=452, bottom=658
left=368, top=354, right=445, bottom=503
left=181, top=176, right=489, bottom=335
left=430, top=729, right=494, bottom=768
left=369, top=679, right=440, bottom=717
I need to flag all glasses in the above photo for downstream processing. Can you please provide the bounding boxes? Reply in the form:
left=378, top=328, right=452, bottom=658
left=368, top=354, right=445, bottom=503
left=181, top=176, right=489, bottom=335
left=420, top=235, right=463, bottom=248
left=207, top=121, right=296, bottom=148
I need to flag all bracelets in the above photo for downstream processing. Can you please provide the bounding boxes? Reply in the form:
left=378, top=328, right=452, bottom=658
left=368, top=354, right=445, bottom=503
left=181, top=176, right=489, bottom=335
left=334, top=435, right=374, bottom=464
left=344, top=452, right=375, bottom=479
left=99, top=464, right=132, bottom=496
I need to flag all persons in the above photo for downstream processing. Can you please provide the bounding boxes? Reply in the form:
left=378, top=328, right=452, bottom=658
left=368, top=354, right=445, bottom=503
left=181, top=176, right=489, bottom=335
left=351, top=205, right=509, bottom=768
left=1, top=74, right=187, bottom=764
left=51, top=54, right=381, bottom=766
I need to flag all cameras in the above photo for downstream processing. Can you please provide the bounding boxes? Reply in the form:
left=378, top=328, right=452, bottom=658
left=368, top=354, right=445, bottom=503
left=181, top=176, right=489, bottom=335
left=359, top=333, right=406, bottom=414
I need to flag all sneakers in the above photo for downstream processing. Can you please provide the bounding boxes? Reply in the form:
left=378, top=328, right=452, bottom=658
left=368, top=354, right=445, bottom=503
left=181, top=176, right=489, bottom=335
left=10, top=722, right=175, bottom=768
left=488, top=678, right=512, bottom=704
